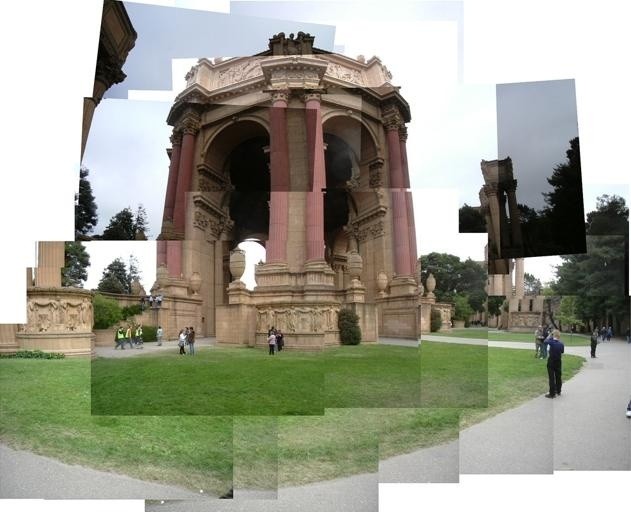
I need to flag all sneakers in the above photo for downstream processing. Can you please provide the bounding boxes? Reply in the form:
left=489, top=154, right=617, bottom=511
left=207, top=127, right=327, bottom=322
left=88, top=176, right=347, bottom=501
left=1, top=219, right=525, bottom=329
left=625, top=410, right=631, bottom=416
left=545, top=391, right=561, bottom=398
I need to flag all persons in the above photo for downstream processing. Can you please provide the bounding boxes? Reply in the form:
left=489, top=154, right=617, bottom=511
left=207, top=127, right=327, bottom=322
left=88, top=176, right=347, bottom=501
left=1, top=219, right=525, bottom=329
left=607, top=326, right=614, bottom=341
left=135, top=327, right=142, bottom=346
left=114, top=326, right=127, bottom=350
left=269, top=327, right=278, bottom=335
left=601, top=326, right=608, bottom=341
left=138, top=324, right=144, bottom=347
left=543, top=329, right=565, bottom=398
left=184, top=327, right=190, bottom=345
left=535, top=325, right=546, bottom=358
left=627, top=328, right=631, bottom=343
left=591, top=331, right=598, bottom=358
left=275, top=329, right=285, bottom=352
left=540, top=324, right=552, bottom=359
left=124, top=326, right=135, bottom=350
left=139, top=294, right=164, bottom=308
left=284, top=34, right=301, bottom=55
left=268, top=331, right=277, bottom=355
left=156, top=325, right=164, bottom=346
left=189, top=326, right=196, bottom=355
left=594, top=326, right=600, bottom=337
left=178, top=328, right=188, bottom=355
